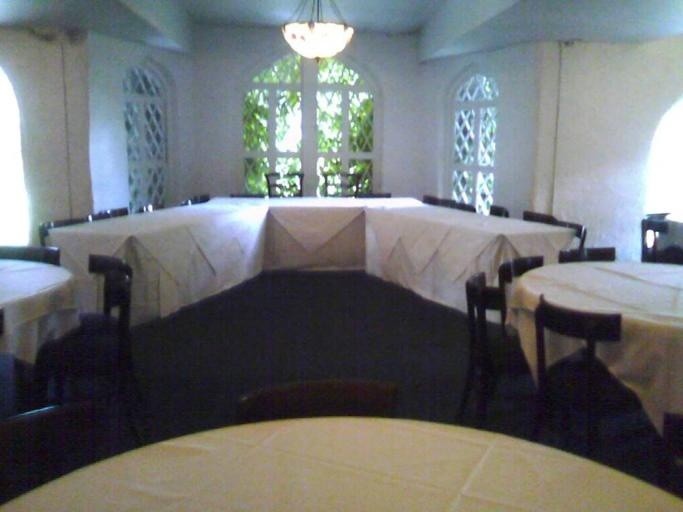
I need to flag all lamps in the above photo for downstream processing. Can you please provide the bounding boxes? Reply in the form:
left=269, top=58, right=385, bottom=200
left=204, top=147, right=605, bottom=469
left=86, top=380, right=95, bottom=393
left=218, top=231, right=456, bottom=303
left=280, top=0, right=353, bottom=64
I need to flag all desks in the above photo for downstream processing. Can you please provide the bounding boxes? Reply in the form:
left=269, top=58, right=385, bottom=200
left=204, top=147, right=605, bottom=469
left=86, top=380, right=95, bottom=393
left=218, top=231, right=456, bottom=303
left=1, top=415, right=682, bottom=510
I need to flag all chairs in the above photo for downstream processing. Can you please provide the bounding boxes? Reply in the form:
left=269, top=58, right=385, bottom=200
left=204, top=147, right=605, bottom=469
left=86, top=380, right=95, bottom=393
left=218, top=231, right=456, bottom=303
left=456, top=213, right=682, bottom=457
left=40, top=194, right=269, bottom=325
left=1, top=246, right=145, bottom=444
left=365, top=194, right=587, bottom=325
left=265, top=173, right=422, bottom=271
left=236, top=382, right=390, bottom=426
